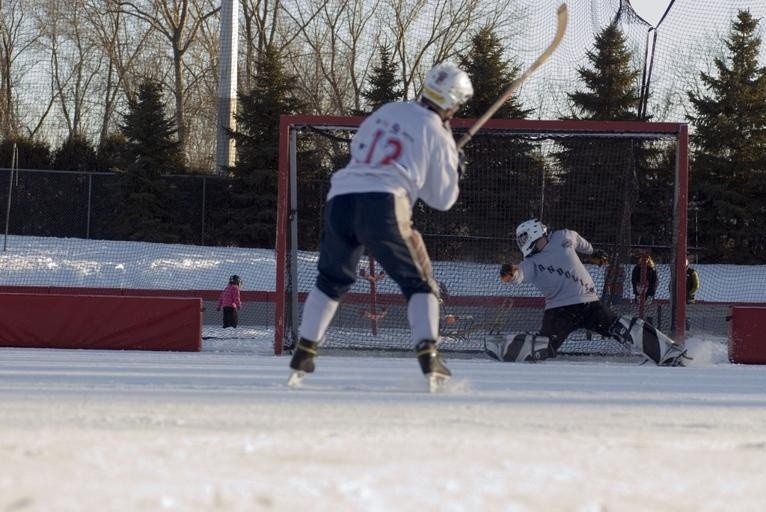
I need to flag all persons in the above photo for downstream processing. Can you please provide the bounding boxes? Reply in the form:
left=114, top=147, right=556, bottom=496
left=285, top=58, right=475, bottom=381
left=498, top=217, right=682, bottom=362
left=602, top=245, right=629, bottom=309
left=668, top=257, right=701, bottom=336
left=631, top=250, right=659, bottom=310
left=215, top=274, right=244, bottom=329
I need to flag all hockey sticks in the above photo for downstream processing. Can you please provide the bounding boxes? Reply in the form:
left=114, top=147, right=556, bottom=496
left=454, top=3, right=567, bottom=151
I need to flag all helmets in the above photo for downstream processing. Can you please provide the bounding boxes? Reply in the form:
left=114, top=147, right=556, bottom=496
left=516, top=218, right=548, bottom=257
left=421, top=62, right=475, bottom=110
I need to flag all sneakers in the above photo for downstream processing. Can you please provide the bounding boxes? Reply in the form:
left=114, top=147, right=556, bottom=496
left=290, top=338, right=318, bottom=374
left=416, top=340, right=452, bottom=379
